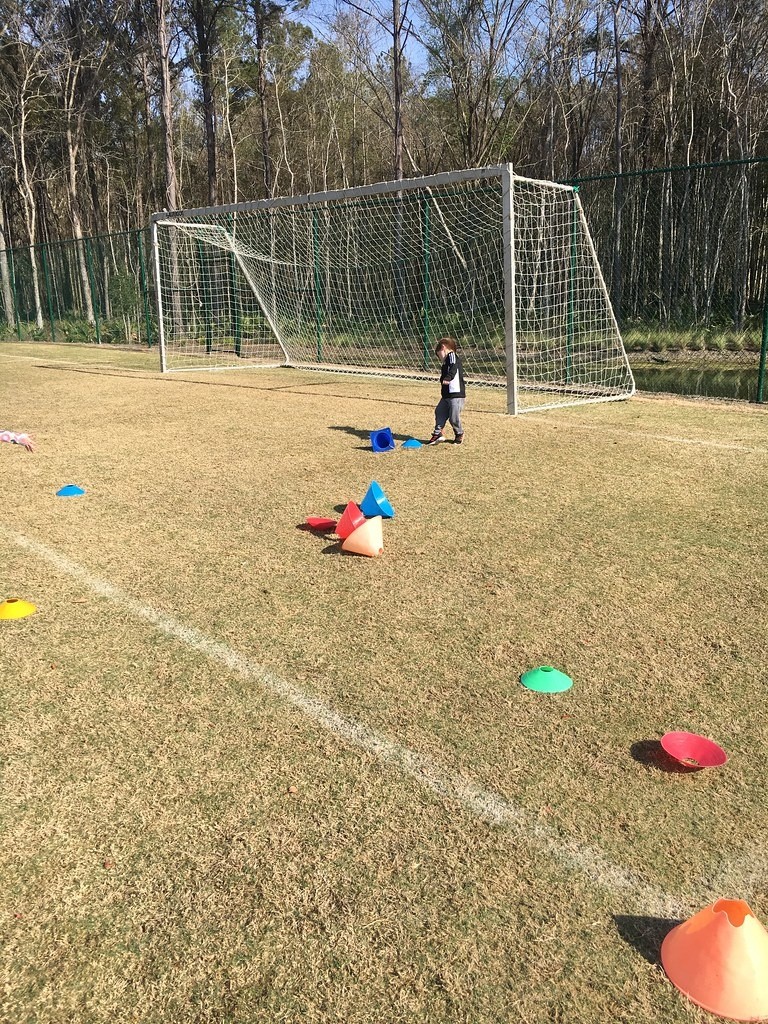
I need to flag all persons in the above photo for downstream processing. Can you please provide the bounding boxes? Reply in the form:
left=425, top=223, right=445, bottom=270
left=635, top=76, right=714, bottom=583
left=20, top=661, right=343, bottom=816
left=429, top=337, right=466, bottom=445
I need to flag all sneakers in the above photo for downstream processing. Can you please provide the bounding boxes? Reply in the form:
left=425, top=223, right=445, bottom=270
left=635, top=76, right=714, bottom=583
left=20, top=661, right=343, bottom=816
left=453, top=433, right=464, bottom=445
left=427, top=432, right=445, bottom=445
